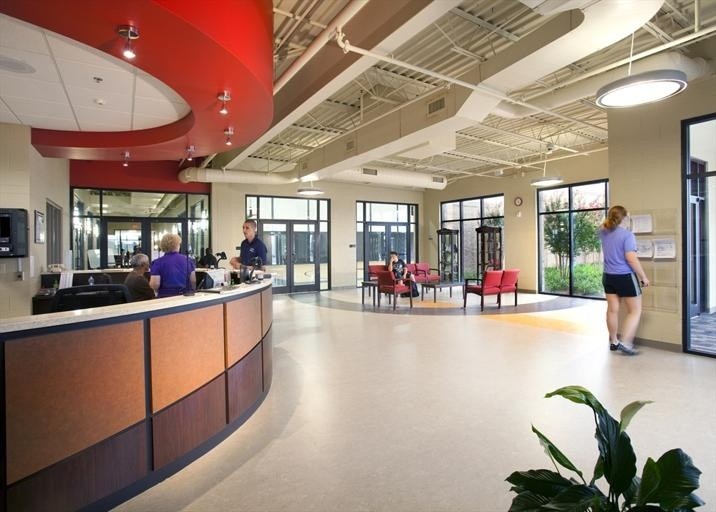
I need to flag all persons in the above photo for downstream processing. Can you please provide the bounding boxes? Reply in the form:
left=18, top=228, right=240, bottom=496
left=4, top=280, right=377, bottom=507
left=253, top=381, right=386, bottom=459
left=388, top=251, right=416, bottom=285
left=229, top=219, right=267, bottom=272
left=149, top=233, right=196, bottom=298
left=123, top=253, right=156, bottom=301
left=199, top=248, right=218, bottom=269
left=598, top=206, right=649, bottom=354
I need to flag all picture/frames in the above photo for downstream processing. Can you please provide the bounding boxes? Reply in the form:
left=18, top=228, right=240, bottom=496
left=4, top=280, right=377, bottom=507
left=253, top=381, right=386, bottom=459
left=34, top=210, right=45, bottom=244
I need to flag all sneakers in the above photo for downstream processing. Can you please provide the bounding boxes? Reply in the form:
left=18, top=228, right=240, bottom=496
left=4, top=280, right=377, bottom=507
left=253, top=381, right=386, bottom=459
left=618, top=342, right=640, bottom=357
left=610, top=340, right=620, bottom=351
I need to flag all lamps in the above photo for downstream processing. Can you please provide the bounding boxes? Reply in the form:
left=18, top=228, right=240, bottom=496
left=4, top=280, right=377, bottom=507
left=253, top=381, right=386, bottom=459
left=530, top=154, right=563, bottom=186
left=122, top=31, right=137, bottom=59
left=297, top=181, right=325, bottom=196
left=219, top=97, right=228, bottom=115
left=596, top=33, right=688, bottom=109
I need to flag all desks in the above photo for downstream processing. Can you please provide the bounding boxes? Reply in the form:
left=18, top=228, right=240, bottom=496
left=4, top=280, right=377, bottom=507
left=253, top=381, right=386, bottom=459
left=421, top=282, right=466, bottom=303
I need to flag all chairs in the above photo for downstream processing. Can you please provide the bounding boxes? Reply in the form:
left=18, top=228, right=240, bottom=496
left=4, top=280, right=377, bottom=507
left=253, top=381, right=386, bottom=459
left=464, top=270, right=503, bottom=312
left=361, top=263, right=440, bottom=310
left=497, top=270, right=520, bottom=307
